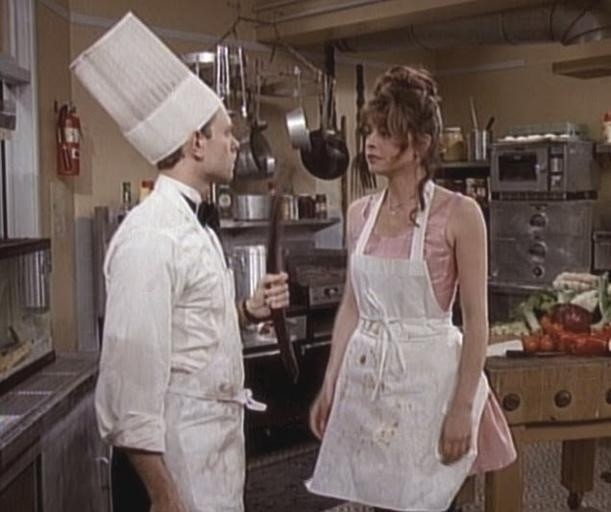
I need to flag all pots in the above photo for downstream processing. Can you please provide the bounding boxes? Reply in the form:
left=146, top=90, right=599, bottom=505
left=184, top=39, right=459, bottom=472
left=233, top=241, right=269, bottom=298
left=233, top=57, right=349, bottom=179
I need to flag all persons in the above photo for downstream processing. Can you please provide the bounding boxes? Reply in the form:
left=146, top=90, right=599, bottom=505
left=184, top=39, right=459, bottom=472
left=302, top=63, right=520, bottom=510
left=88, top=99, right=293, bottom=510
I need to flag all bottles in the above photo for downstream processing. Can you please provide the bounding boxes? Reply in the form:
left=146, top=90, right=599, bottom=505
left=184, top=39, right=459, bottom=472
left=438, top=126, right=465, bottom=162
left=298, top=194, right=327, bottom=219
left=118, top=182, right=131, bottom=224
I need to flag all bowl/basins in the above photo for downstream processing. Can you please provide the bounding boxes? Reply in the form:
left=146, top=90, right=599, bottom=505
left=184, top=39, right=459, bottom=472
left=232, top=193, right=273, bottom=222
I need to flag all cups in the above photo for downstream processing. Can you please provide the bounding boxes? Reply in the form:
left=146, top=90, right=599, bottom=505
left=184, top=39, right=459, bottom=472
left=469, top=128, right=491, bottom=161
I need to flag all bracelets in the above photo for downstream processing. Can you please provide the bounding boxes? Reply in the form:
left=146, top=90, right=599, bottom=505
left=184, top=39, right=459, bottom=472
left=240, top=296, right=264, bottom=325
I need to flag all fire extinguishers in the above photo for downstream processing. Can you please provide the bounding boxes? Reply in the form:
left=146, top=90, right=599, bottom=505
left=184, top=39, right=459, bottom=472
left=57, top=103, right=82, bottom=177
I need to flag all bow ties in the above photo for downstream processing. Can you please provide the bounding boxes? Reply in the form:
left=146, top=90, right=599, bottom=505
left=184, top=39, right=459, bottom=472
left=196, top=199, right=221, bottom=230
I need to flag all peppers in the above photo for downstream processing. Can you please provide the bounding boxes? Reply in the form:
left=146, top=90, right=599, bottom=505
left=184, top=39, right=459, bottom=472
left=550, top=276, right=611, bottom=356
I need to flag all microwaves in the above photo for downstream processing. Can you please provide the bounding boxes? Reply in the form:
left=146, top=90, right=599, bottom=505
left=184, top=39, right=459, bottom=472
left=491, top=138, right=595, bottom=192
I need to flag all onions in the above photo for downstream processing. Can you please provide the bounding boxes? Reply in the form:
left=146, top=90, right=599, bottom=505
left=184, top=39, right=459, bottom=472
left=526, top=336, right=554, bottom=354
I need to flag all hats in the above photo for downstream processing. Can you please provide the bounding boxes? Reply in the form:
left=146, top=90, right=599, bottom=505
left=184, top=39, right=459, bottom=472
left=66, top=7, right=225, bottom=167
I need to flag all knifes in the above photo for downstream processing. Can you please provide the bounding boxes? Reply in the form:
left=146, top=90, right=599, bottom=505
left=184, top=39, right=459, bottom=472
left=267, top=280, right=300, bottom=387
